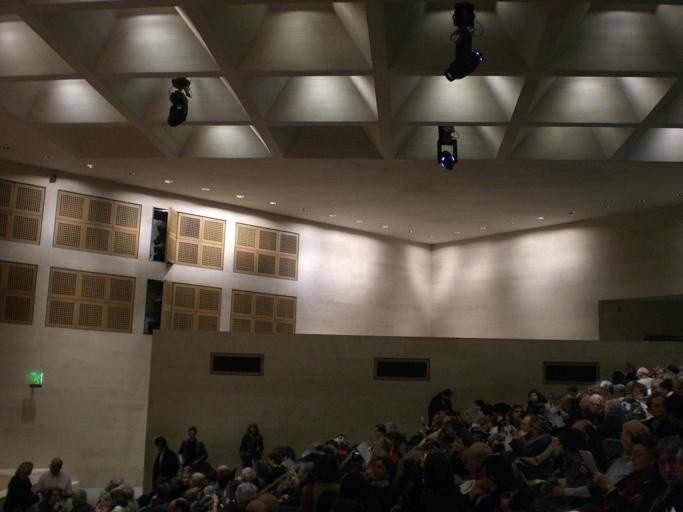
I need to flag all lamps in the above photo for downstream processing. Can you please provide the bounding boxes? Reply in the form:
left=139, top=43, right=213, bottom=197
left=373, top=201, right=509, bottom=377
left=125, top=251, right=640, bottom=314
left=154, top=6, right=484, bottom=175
left=434, top=125, right=458, bottom=169
left=167, top=75, right=191, bottom=126
left=443, top=0, right=484, bottom=82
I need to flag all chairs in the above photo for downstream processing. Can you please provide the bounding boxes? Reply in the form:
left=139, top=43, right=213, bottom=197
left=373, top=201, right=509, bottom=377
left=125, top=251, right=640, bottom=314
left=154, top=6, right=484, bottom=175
left=136, top=416, right=637, bottom=512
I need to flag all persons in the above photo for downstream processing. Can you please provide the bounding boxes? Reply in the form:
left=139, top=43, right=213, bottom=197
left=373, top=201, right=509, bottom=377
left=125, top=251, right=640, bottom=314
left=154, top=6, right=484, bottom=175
left=285, top=364, right=682, bottom=511
left=138, top=421, right=296, bottom=511
left=6, top=457, right=141, bottom=512
